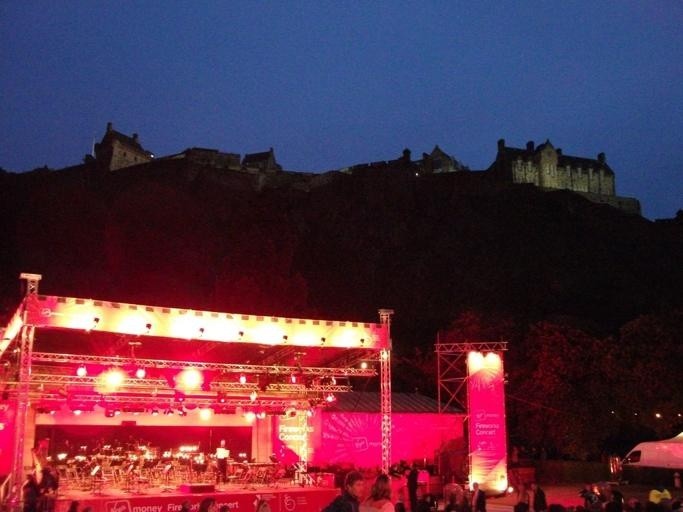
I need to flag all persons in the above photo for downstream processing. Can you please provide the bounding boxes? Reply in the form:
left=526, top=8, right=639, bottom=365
left=67, top=500, right=92, bottom=512
left=649, top=481, right=672, bottom=512
left=603, top=487, right=625, bottom=512
left=514, top=483, right=546, bottom=512
left=22, top=467, right=58, bottom=511
left=211, top=459, right=212, bottom=460
left=255, top=500, right=271, bottom=512
left=179, top=498, right=230, bottom=512
left=320, top=462, right=486, bottom=512
left=210, top=439, right=230, bottom=484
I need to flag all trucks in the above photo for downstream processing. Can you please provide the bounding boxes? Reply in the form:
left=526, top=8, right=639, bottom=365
left=616, top=439, right=682, bottom=489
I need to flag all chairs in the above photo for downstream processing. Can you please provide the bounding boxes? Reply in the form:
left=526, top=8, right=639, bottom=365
left=56, top=451, right=315, bottom=492
